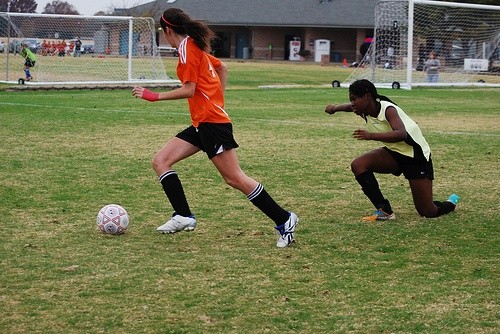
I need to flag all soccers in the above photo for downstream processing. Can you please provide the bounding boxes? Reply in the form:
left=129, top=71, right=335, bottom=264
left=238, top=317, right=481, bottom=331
left=97, top=203, right=130, bottom=236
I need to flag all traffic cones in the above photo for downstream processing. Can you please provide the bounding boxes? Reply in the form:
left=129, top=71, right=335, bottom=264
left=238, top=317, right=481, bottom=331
left=342, top=58, right=348, bottom=66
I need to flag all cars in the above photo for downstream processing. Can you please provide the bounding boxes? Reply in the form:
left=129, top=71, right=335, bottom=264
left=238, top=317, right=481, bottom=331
left=0, top=39, right=94, bottom=54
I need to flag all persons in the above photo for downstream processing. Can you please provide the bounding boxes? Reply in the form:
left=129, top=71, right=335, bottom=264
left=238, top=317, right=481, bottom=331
left=0, top=36, right=83, bottom=58
left=423, top=50, right=441, bottom=82
left=131, top=8, right=301, bottom=248
left=105, top=47, right=111, bottom=54
left=325, top=78, right=461, bottom=222
left=21, top=42, right=37, bottom=82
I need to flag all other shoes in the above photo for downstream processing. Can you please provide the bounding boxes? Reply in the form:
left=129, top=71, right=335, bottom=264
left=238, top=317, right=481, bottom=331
left=25, top=75, right=33, bottom=81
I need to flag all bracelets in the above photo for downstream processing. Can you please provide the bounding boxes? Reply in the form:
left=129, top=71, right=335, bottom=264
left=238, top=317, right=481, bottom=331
left=331, top=106, right=336, bottom=113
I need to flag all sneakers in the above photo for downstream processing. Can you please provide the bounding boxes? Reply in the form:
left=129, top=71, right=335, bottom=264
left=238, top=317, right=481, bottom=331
left=447, top=194, right=459, bottom=205
left=157, top=215, right=197, bottom=233
left=362, top=209, right=395, bottom=222
left=274, top=213, right=300, bottom=247
